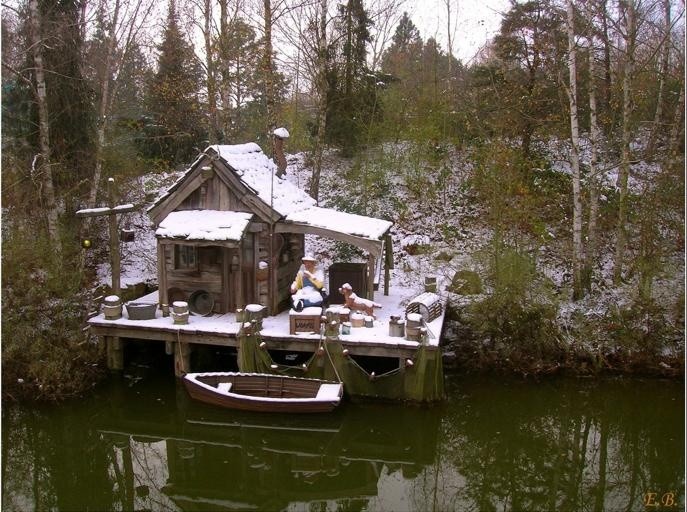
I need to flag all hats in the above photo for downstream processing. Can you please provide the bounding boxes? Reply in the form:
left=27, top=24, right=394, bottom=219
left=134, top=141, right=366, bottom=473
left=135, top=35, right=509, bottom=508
left=302, top=252, right=316, bottom=261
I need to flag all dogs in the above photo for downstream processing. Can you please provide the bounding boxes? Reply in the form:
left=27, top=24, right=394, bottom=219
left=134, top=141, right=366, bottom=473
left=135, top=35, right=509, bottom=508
left=338, top=282, right=384, bottom=321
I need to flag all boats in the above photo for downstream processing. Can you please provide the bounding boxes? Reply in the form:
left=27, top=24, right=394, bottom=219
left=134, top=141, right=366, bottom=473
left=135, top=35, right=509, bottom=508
left=185, top=407, right=348, bottom=440
left=181, top=369, right=344, bottom=414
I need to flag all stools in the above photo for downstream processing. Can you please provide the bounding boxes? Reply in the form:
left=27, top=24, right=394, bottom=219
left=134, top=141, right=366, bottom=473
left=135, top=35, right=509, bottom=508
left=289, top=307, right=322, bottom=335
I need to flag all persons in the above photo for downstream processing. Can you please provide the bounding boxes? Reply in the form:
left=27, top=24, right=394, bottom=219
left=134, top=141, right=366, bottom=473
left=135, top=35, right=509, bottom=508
left=289, top=253, right=328, bottom=313
